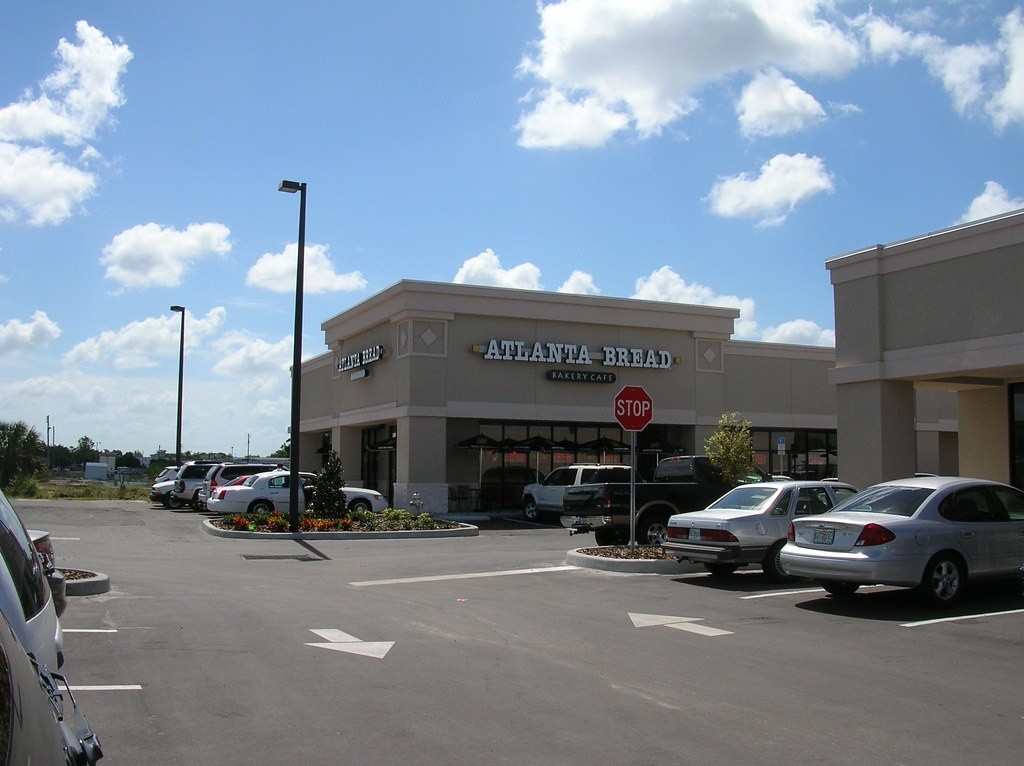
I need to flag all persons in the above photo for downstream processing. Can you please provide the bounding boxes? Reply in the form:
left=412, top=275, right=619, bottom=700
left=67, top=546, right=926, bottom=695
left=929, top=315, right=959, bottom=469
left=271, top=463, right=285, bottom=486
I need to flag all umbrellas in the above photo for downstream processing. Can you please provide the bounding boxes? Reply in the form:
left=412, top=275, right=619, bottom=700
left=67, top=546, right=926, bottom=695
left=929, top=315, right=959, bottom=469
left=455, top=434, right=637, bottom=489
left=637, top=442, right=688, bottom=467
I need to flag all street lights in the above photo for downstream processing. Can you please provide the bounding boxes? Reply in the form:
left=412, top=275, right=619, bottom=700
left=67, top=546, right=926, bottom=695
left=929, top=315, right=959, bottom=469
left=170, top=305, right=185, bottom=464
left=280, top=180, right=307, bottom=531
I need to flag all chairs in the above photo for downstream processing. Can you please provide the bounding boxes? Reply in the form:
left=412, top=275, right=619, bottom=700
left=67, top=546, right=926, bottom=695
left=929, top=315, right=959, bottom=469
left=803, top=499, right=828, bottom=514
left=957, top=497, right=989, bottom=522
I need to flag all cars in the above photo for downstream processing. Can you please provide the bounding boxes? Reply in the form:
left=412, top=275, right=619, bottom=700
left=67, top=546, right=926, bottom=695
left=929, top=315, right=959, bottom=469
left=481, top=464, right=546, bottom=513
left=520, top=464, right=631, bottom=522
left=206, top=469, right=391, bottom=516
left=167, top=459, right=221, bottom=509
left=779, top=472, right=1024, bottom=610
left=149, top=466, right=180, bottom=507
left=196, top=462, right=290, bottom=506
left=0, top=488, right=107, bottom=766
left=663, top=476, right=860, bottom=580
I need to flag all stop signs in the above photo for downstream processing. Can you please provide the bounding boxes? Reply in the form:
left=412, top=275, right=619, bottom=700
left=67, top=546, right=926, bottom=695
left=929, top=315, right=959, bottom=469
left=614, top=386, right=654, bottom=433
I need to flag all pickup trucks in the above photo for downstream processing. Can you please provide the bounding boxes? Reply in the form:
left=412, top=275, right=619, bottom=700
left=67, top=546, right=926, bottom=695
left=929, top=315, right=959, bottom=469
left=561, top=456, right=774, bottom=546
